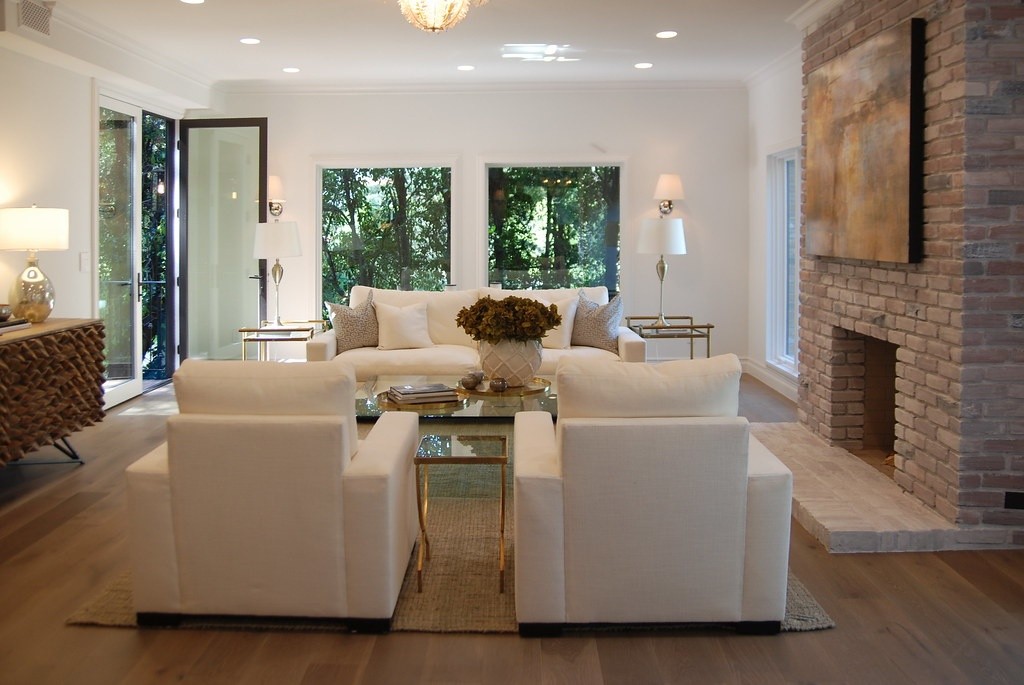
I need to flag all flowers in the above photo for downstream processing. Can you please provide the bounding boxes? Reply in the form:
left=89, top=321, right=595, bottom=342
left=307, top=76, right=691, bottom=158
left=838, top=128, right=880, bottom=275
left=456, top=293, right=563, bottom=347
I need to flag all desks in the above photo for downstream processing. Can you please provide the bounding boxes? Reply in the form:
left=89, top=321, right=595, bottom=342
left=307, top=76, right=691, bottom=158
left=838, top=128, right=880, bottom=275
left=0, top=318, right=107, bottom=465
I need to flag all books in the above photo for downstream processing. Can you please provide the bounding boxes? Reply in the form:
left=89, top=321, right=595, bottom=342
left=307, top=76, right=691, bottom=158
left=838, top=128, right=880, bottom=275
left=387, top=383, right=459, bottom=405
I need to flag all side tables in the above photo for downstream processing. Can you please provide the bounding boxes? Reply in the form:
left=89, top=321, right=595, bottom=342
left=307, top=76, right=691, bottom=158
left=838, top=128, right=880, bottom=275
left=239, top=320, right=329, bottom=362
left=626, top=316, right=714, bottom=361
left=413, top=435, right=508, bottom=594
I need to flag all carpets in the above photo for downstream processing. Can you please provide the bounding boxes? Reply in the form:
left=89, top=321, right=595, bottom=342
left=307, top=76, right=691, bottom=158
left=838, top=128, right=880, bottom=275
left=70, top=419, right=837, bottom=633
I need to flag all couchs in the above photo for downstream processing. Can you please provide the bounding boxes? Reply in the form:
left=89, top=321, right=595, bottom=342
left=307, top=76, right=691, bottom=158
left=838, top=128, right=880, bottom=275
left=514, top=356, right=793, bottom=636
left=127, top=411, right=417, bottom=632
left=308, top=285, right=646, bottom=379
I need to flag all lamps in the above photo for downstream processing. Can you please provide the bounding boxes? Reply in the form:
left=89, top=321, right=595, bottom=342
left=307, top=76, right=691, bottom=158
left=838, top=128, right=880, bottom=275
left=640, top=218, right=688, bottom=331
left=398, top=1, right=471, bottom=33
left=251, top=220, right=300, bottom=335
left=1, top=205, right=68, bottom=322
left=651, top=174, right=683, bottom=213
left=265, top=175, right=285, bottom=215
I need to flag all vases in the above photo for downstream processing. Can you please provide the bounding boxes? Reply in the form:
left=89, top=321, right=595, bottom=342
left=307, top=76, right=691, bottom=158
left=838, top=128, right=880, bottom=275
left=476, top=338, right=544, bottom=386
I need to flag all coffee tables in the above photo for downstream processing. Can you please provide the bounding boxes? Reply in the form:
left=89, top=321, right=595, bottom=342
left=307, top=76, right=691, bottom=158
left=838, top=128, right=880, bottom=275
left=355, top=374, right=558, bottom=441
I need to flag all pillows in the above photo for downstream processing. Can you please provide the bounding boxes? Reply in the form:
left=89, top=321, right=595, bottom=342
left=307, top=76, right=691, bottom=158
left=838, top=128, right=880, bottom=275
left=370, top=299, right=437, bottom=350
left=325, top=292, right=378, bottom=355
left=571, top=288, right=623, bottom=354
left=174, top=359, right=358, bottom=459
left=530, top=295, right=577, bottom=350
left=556, top=354, right=742, bottom=443
